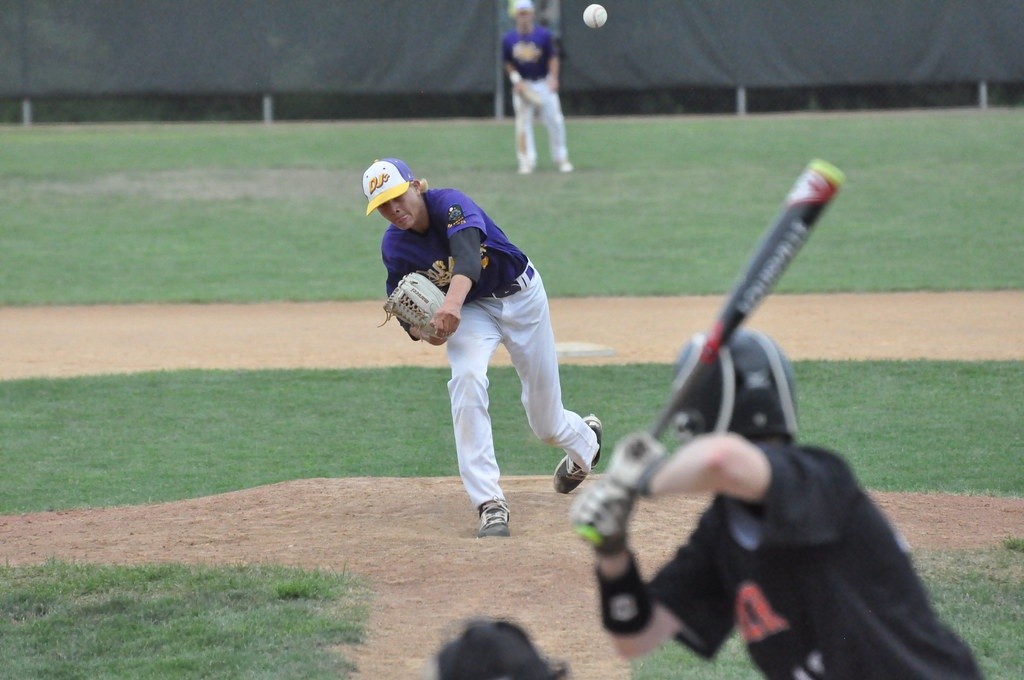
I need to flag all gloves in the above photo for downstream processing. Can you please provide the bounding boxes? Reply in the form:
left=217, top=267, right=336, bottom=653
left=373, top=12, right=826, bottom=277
left=570, top=475, right=634, bottom=554
left=609, top=430, right=669, bottom=497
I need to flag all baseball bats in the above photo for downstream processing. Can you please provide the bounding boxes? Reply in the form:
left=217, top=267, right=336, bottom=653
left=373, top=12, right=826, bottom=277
left=570, top=155, right=848, bottom=552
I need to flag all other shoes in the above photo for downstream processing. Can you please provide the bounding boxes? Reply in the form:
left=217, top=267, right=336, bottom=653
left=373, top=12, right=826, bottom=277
left=519, top=164, right=534, bottom=174
left=553, top=158, right=572, bottom=173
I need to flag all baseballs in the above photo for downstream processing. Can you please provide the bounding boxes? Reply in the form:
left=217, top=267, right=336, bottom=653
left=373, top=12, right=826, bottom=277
left=581, top=3, right=609, bottom=30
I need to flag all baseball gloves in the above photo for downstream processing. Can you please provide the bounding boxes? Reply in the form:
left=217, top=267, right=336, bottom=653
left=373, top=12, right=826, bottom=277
left=375, top=271, right=461, bottom=341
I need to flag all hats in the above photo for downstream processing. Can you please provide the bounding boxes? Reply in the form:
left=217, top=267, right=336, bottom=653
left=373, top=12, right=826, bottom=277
left=507, top=0, right=535, bottom=16
left=362, top=158, right=413, bottom=216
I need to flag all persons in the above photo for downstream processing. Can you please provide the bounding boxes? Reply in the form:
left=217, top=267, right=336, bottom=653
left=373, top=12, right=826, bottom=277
left=499, top=0, right=574, bottom=173
left=567, top=325, right=985, bottom=680
left=360, top=156, right=601, bottom=536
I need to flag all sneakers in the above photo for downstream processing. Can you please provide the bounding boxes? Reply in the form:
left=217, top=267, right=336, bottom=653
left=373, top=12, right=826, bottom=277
left=553, top=414, right=602, bottom=494
left=478, top=495, right=510, bottom=539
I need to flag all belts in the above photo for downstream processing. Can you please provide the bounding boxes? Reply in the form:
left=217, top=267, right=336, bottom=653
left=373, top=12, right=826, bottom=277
left=482, top=266, right=533, bottom=298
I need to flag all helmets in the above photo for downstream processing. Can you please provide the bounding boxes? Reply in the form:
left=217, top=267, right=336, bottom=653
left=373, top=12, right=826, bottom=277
left=674, top=329, right=799, bottom=446
